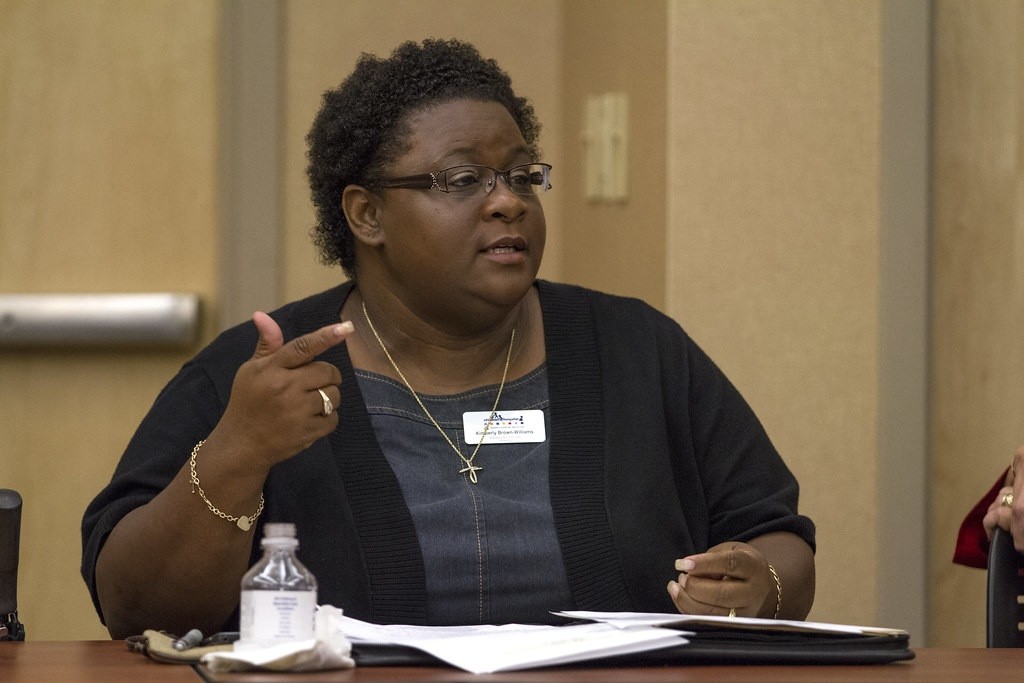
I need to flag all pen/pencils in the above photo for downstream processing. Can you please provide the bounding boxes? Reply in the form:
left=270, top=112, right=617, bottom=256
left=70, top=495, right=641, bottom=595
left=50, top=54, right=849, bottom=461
left=176, top=628, right=203, bottom=650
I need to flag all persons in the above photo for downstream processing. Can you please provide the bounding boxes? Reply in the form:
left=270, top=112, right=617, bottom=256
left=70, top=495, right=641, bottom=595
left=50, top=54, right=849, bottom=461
left=951, top=445, right=1024, bottom=569
left=81, top=36, right=816, bottom=639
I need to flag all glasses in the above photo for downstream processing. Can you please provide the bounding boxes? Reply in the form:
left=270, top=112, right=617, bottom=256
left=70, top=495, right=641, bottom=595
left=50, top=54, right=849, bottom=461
left=369, top=162, right=552, bottom=200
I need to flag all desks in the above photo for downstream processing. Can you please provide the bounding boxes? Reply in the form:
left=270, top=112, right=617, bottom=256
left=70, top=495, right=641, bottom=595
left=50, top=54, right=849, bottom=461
left=0, top=640, right=1024, bottom=683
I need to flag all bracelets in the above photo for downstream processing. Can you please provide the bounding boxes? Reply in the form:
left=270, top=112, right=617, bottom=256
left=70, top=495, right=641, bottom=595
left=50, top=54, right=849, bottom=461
left=769, top=565, right=783, bottom=619
left=190, top=440, right=264, bottom=531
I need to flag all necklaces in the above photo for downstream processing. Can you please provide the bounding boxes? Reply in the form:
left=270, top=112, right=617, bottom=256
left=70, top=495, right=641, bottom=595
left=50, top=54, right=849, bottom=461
left=362, top=300, right=514, bottom=484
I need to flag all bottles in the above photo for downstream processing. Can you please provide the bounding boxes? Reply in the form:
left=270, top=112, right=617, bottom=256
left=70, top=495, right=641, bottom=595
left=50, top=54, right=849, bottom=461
left=241, top=524, right=317, bottom=653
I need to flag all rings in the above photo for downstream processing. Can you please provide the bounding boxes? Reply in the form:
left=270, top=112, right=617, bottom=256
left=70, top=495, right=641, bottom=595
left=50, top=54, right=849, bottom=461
left=727, top=608, right=737, bottom=617
left=1001, top=494, right=1013, bottom=507
left=318, top=389, right=333, bottom=416
